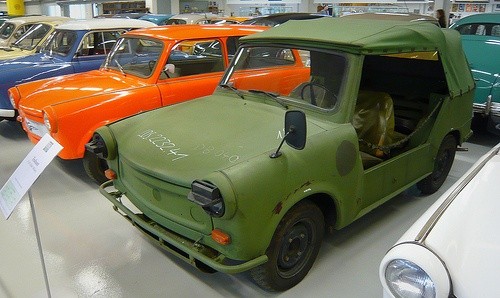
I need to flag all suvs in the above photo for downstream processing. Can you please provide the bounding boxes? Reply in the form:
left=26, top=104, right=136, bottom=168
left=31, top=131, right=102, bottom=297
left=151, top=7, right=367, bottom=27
left=6, top=24, right=310, bottom=188
left=0, top=16, right=159, bottom=127
left=84, top=10, right=477, bottom=293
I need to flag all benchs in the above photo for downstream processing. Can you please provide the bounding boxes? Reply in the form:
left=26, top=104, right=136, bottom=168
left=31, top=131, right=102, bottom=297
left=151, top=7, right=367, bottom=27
left=387, top=88, right=429, bottom=149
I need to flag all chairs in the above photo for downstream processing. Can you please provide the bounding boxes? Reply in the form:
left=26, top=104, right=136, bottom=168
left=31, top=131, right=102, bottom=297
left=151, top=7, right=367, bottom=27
left=353, top=90, right=396, bottom=166
left=288, top=74, right=330, bottom=107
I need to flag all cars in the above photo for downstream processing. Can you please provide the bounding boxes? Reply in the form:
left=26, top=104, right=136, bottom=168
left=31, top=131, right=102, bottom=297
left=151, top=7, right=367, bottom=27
left=448, top=9, right=500, bottom=138
left=379, top=141, right=500, bottom=298
left=93, top=12, right=333, bottom=65
